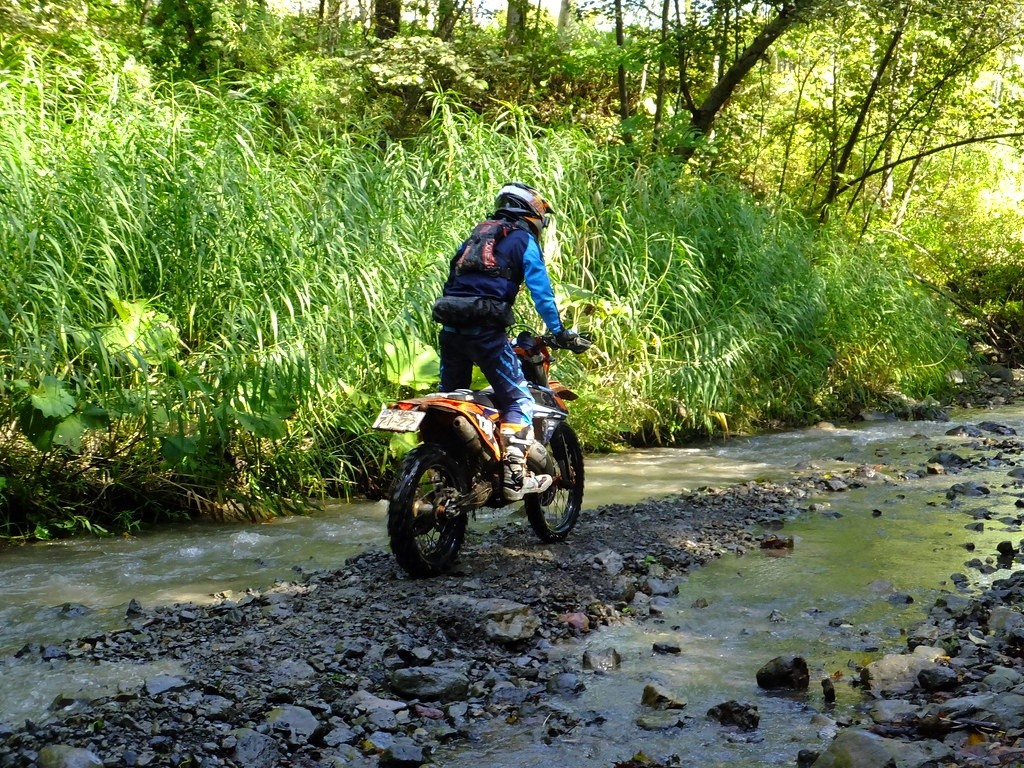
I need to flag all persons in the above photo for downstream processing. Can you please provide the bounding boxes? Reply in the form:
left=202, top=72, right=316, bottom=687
left=432, top=182, right=578, bottom=500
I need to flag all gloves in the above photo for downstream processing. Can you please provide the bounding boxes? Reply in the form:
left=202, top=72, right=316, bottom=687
left=554, top=329, right=580, bottom=349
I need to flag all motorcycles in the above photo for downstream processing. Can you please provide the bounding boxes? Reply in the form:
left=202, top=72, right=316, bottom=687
left=371, top=322, right=597, bottom=580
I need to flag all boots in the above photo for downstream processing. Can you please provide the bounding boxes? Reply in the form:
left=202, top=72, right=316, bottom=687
left=500, top=423, right=552, bottom=500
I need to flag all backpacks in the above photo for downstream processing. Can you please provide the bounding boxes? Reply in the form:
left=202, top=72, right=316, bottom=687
left=455, top=217, right=512, bottom=280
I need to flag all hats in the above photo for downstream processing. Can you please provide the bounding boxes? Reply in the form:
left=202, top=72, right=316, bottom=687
left=494, top=182, right=556, bottom=239
